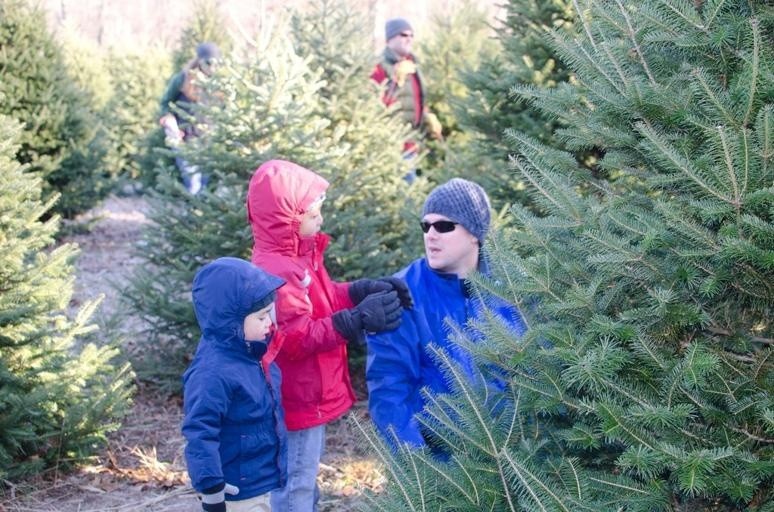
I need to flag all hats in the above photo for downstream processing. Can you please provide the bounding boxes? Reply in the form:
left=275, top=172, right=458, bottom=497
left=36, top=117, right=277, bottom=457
left=198, top=43, right=221, bottom=60
left=424, top=178, right=491, bottom=245
left=385, top=19, right=411, bottom=40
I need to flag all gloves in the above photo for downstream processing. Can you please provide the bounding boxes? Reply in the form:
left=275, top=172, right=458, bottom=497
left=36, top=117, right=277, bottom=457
left=332, top=290, right=404, bottom=344
left=198, top=481, right=239, bottom=512
left=349, top=277, right=414, bottom=311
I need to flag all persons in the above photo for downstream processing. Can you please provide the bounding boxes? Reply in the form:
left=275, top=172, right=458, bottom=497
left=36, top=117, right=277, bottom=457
left=180, top=256, right=288, bottom=512
left=364, top=177, right=550, bottom=468
left=161, top=44, right=234, bottom=197
left=248, top=158, right=413, bottom=512
left=366, top=18, right=443, bottom=185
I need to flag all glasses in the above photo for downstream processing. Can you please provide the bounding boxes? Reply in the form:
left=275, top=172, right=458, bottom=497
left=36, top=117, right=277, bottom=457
left=419, top=222, right=458, bottom=233
